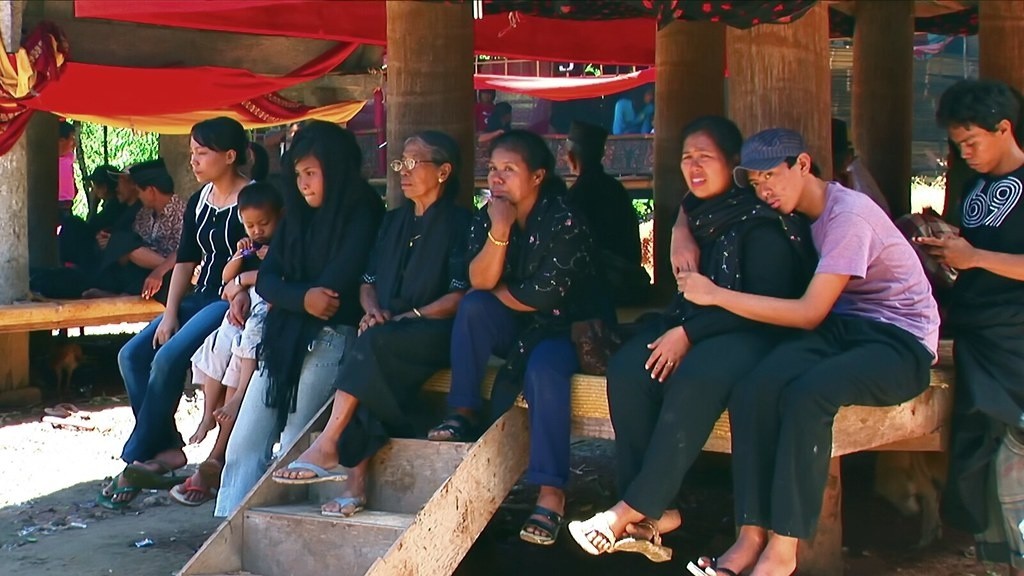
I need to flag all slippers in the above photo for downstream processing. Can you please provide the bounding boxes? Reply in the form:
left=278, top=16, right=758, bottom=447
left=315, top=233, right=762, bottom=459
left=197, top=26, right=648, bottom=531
left=427, top=414, right=479, bottom=442
left=322, top=496, right=363, bottom=517
left=568, top=511, right=615, bottom=556
left=99, top=474, right=142, bottom=510
left=122, top=459, right=191, bottom=489
left=520, top=505, right=565, bottom=545
left=170, top=478, right=216, bottom=507
left=271, top=460, right=348, bottom=484
left=42, top=403, right=95, bottom=430
left=686, top=554, right=738, bottom=576
left=614, top=520, right=673, bottom=563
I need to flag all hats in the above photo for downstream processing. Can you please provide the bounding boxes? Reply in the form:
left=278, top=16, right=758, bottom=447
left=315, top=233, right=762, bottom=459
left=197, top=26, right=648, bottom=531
left=568, top=120, right=608, bottom=151
left=733, top=128, right=806, bottom=188
left=84, top=166, right=119, bottom=182
left=832, top=117, right=854, bottom=146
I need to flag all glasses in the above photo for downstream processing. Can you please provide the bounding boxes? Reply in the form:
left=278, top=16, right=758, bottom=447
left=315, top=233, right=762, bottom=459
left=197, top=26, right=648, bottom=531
left=391, top=158, right=442, bottom=173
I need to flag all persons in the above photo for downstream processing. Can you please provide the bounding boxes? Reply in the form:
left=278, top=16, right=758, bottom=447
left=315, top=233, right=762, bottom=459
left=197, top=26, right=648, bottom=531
left=57, top=120, right=187, bottom=306
left=97, top=116, right=270, bottom=508
left=169, top=74, right=1024, bottom=576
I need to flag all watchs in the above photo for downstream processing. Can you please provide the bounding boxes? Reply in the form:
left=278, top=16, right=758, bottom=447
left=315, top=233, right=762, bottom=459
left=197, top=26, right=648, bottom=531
left=234, top=273, right=245, bottom=290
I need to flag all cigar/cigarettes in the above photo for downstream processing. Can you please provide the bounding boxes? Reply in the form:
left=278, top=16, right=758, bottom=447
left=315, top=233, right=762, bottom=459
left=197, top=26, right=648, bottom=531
left=145, top=290, right=149, bottom=294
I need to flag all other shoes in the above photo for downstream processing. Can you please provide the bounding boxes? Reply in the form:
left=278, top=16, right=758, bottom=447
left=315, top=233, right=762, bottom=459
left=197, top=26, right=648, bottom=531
left=82, top=289, right=101, bottom=298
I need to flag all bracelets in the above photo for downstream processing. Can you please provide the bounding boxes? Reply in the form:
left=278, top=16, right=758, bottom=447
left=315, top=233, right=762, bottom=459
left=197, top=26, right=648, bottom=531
left=488, top=231, right=510, bottom=246
left=413, top=307, right=422, bottom=318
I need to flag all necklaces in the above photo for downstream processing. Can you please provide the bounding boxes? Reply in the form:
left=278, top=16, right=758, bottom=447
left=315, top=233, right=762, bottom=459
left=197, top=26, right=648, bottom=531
left=212, top=183, right=232, bottom=221
